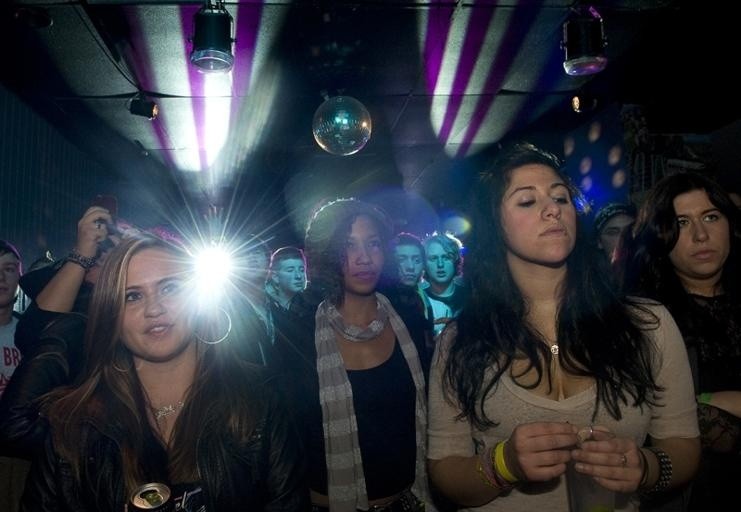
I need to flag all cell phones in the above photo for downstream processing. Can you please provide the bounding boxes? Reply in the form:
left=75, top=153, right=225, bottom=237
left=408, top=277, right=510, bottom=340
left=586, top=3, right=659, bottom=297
left=94, top=195, right=117, bottom=235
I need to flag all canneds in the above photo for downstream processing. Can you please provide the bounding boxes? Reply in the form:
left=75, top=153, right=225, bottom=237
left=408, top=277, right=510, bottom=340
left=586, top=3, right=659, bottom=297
left=127, top=482, right=177, bottom=512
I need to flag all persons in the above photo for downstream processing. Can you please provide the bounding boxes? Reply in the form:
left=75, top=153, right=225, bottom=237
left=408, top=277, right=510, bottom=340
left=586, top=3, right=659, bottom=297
left=0, top=145, right=741, bottom=512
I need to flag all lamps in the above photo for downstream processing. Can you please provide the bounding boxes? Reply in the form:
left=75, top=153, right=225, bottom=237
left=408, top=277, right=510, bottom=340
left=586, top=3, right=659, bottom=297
left=553, top=7, right=611, bottom=87
left=570, top=93, right=598, bottom=120
left=187, top=8, right=237, bottom=78
left=128, top=85, right=163, bottom=123
